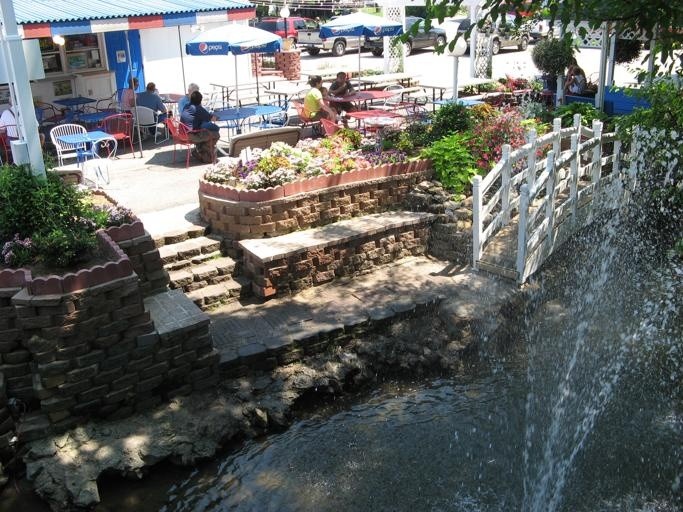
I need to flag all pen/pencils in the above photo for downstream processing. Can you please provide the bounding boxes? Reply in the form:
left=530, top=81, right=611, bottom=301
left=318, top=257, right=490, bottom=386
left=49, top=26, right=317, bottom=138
left=261, top=16, right=321, bottom=44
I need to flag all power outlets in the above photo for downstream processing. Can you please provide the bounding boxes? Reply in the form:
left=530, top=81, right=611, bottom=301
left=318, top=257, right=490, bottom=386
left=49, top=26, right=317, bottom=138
left=365, top=17, right=446, bottom=57
left=296, top=28, right=365, bottom=56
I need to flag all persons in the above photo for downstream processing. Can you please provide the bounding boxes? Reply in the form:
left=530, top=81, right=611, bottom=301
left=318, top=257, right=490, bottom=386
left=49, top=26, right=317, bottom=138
left=328, top=72, right=356, bottom=128
left=564, top=67, right=585, bottom=94
left=0, top=96, right=39, bottom=162
left=179, top=91, right=220, bottom=163
left=178, top=84, right=219, bottom=131
left=121, top=78, right=143, bottom=135
left=136, top=83, right=167, bottom=137
left=304, top=76, right=334, bottom=136
left=318, top=87, right=341, bottom=121
left=566, top=58, right=585, bottom=85
left=33, top=98, right=46, bottom=149
left=310, top=17, right=321, bottom=28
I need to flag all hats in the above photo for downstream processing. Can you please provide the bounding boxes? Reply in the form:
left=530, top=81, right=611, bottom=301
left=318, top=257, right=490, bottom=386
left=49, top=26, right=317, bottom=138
left=458, top=18, right=529, bottom=55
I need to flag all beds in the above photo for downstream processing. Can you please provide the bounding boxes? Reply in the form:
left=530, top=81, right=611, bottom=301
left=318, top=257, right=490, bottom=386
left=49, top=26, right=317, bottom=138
left=318, top=12, right=403, bottom=111
left=185, top=22, right=282, bottom=128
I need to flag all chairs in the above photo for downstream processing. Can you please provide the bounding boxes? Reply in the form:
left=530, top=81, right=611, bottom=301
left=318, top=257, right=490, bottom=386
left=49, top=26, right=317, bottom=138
left=2, top=85, right=127, bottom=167
left=167, top=119, right=215, bottom=167
left=51, top=124, right=90, bottom=169
left=192, top=149, right=219, bottom=164
left=102, top=113, right=135, bottom=159
left=131, top=105, right=167, bottom=145
left=214, top=67, right=600, bottom=169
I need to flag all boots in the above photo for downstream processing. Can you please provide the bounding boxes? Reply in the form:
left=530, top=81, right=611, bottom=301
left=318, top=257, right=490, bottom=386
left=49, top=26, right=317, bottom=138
left=529, top=20, right=561, bottom=40
